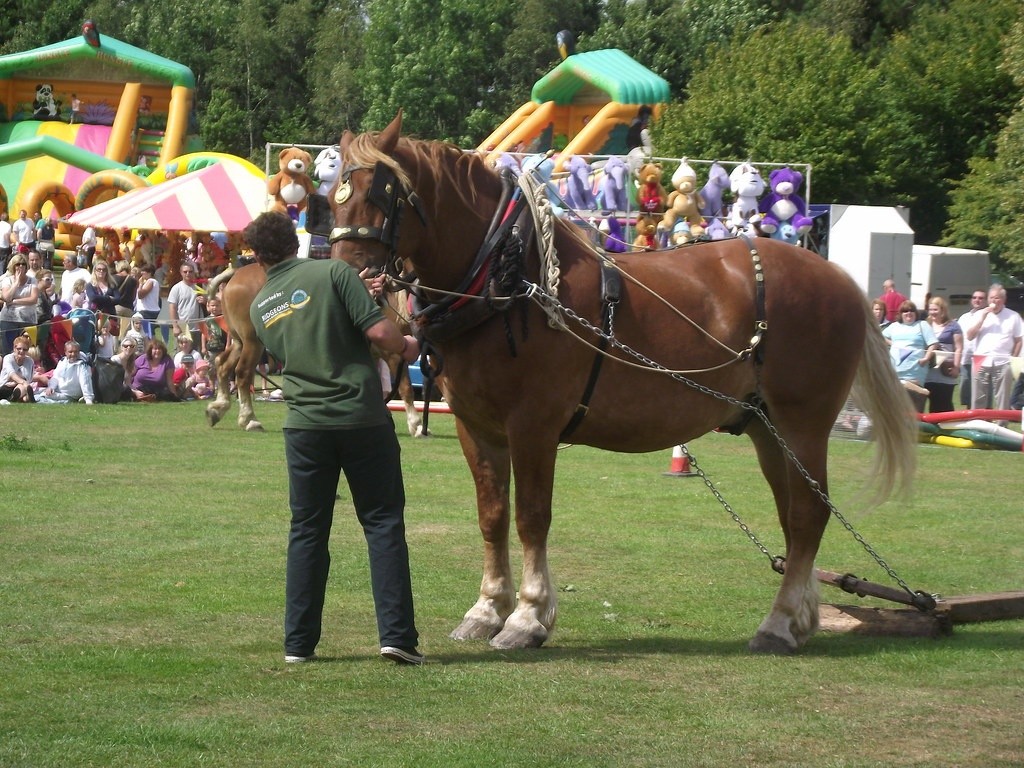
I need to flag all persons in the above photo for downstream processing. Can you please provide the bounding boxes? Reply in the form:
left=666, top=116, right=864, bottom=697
left=242, top=213, right=423, bottom=665
left=851, top=280, right=1024, bottom=429
left=626, top=105, right=653, bottom=149
left=0, top=210, right=359, bottom=406
left=69, top=94, right=84, bottom=125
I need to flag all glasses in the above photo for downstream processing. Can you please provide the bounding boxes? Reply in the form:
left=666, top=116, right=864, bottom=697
left=23, top=335, right=30, bottom=338
left=902, top=309, right=915, bottom=312
left=183, top=271, right=193, bottom=273
left=96, top=268, right=108, bottom=272
left=15, top=263, right=26, bottom=266
left=41, top=277, right=53, bottom=282
left=122, top=345, right=135, bottom=348
left=16, top=348, right=28, bottom=352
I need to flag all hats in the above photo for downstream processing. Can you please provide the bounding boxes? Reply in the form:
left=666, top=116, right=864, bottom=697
left=181, top=354, right=194, bottom=363
left=196, top=360, right=211, bottom=375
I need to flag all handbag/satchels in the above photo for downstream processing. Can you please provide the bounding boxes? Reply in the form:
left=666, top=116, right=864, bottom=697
left=941, top=362, right=960, bottom=377
left=92, top=357, right=125, bottom=404
left=206, top=335, right=225, bottom=351
left=109, top=318, right=120, bottom=336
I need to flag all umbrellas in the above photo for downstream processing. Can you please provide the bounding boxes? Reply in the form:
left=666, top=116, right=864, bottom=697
left=58, top=162, right=276, bottom=234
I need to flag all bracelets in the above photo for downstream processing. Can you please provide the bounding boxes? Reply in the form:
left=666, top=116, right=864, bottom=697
left=397, top=336, right=408, bottom=355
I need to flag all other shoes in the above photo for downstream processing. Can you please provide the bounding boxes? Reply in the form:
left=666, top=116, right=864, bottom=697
left=27, top=385, right=35, bottom=403
left=7, top=388, right=21, bottom=402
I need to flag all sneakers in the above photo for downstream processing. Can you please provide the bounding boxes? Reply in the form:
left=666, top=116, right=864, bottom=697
left=285, top=652, right=318, bottom=663
left=380, top=647, right=425, bottom=664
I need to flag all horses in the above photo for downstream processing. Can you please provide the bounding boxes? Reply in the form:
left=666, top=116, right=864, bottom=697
left=205, top=263, right=434, bottom=441
left=327, top=107, right=919, bottom=655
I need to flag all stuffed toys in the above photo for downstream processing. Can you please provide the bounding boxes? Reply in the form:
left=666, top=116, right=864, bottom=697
left=266, top=143, right=812, bottom=246
